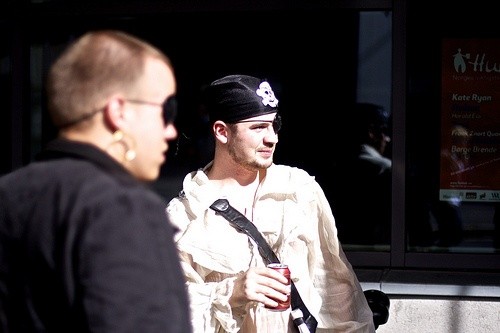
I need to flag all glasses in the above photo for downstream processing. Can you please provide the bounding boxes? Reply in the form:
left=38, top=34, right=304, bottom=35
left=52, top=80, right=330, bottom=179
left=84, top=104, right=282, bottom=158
left=123, top=95, right=177, bottom=126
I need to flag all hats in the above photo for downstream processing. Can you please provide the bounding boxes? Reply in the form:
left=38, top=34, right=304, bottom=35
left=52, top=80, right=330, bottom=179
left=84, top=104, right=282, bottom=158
left=207, top=74, right=278, bottom=125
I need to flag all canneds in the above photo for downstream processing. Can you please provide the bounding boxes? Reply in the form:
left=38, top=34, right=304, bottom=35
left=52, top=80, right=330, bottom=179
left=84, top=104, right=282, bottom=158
left=263, top=263, right=291, bottom=312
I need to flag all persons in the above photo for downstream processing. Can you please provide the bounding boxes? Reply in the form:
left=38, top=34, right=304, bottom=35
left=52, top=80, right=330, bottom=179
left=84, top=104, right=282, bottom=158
left=335, top=102, right=468, bottom=252
left=0, top=28, right=194, bottom=333
left=165, top=74, right=376, bottom=332
